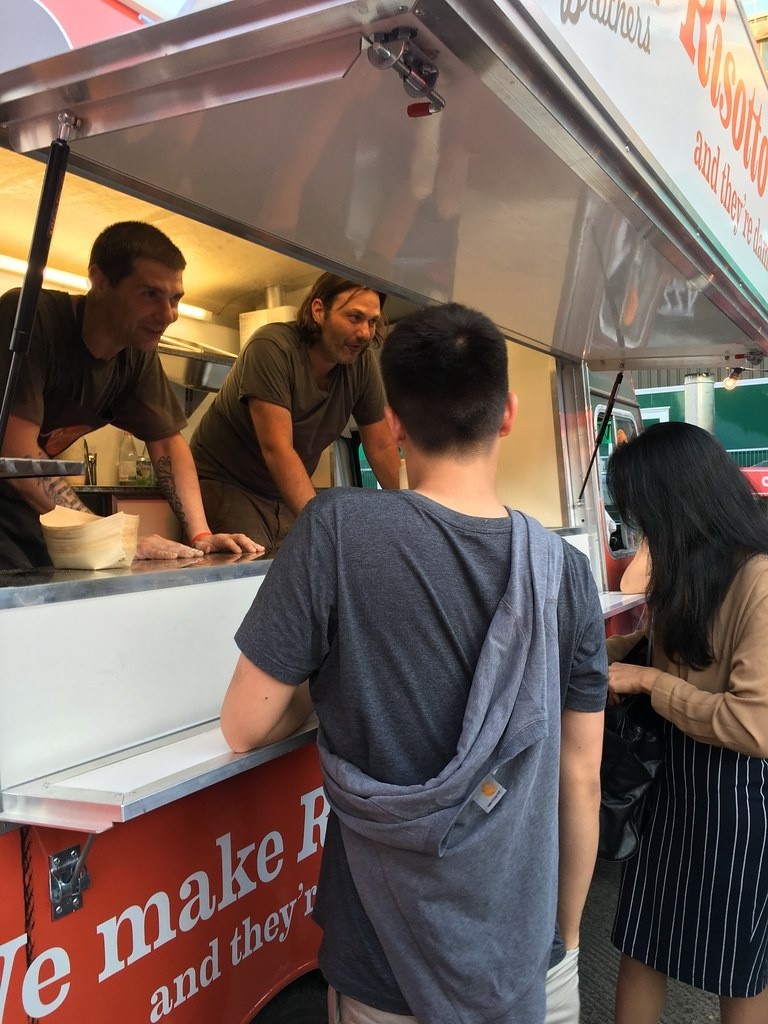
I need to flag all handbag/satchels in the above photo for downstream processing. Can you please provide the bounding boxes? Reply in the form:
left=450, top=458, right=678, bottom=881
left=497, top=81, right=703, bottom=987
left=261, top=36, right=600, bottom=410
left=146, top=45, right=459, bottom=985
left=595, top=633, right=667, bottom=863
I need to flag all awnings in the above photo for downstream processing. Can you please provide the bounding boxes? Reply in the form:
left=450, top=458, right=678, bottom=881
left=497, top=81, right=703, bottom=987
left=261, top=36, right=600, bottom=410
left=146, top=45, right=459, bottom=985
left=0, top=0, right=768, bottom=369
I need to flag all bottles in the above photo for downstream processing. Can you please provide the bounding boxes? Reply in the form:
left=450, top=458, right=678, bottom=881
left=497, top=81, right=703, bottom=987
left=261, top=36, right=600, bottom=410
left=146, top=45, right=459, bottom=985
left=118, top=431, right=141, bottom=487
left=143, top=443, right=153, bottom=459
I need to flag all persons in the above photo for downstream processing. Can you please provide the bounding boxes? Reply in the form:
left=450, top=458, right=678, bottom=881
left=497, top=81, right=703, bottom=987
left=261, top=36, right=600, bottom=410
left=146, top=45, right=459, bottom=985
left=188, top=271, right=402, bottom=553
left=600, top=420, right=768, bottom=1024
left=220, top=303, right=610, bottom=1024
left=0, top=218, right=267, bottom=570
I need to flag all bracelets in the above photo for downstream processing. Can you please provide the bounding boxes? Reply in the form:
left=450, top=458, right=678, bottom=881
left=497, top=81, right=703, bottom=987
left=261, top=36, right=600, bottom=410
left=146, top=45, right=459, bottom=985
left=188, top=532, right=213, bottom=548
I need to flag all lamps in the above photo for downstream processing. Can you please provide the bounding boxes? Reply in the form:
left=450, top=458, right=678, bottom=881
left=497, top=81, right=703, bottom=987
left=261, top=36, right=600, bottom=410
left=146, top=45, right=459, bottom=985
left=0, top=252, right=214, bottom=324
